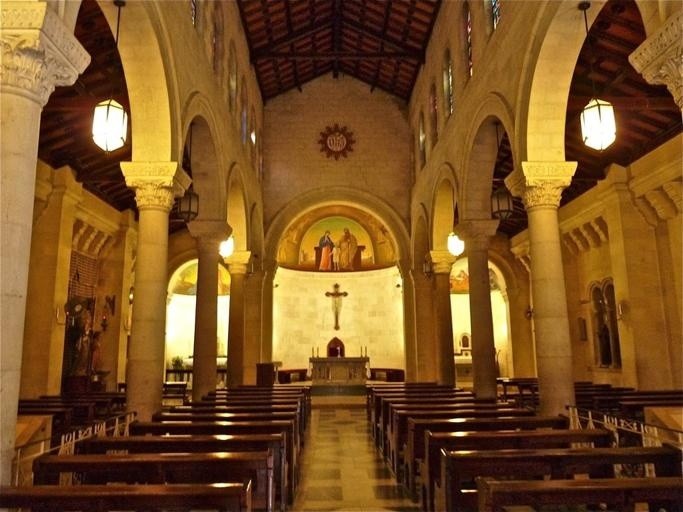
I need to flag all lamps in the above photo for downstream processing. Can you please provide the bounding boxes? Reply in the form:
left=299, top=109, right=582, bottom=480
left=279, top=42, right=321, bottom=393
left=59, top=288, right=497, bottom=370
left=574, top=0, right=616, bottom=154
left=91, top=0, right=128, bottom=152
left=447, top=201, right=464, bottom=258
left=177, top=122, right=200, bottom=222
left=489, top=121, right=512, bottom=223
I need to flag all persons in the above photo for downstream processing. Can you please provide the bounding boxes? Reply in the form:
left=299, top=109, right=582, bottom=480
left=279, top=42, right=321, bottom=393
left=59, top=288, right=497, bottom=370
left=318, top=230, right=333, bottom=271
left=328, top=240, right=340, bottom=271
left=340, top=227, right=356, bottom=271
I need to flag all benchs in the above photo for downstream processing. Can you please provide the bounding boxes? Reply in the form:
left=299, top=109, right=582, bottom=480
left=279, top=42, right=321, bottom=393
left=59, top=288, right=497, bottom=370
left=368, top=367, right=404, bottom=383
left=277, top=368, right=308, bottom=384
